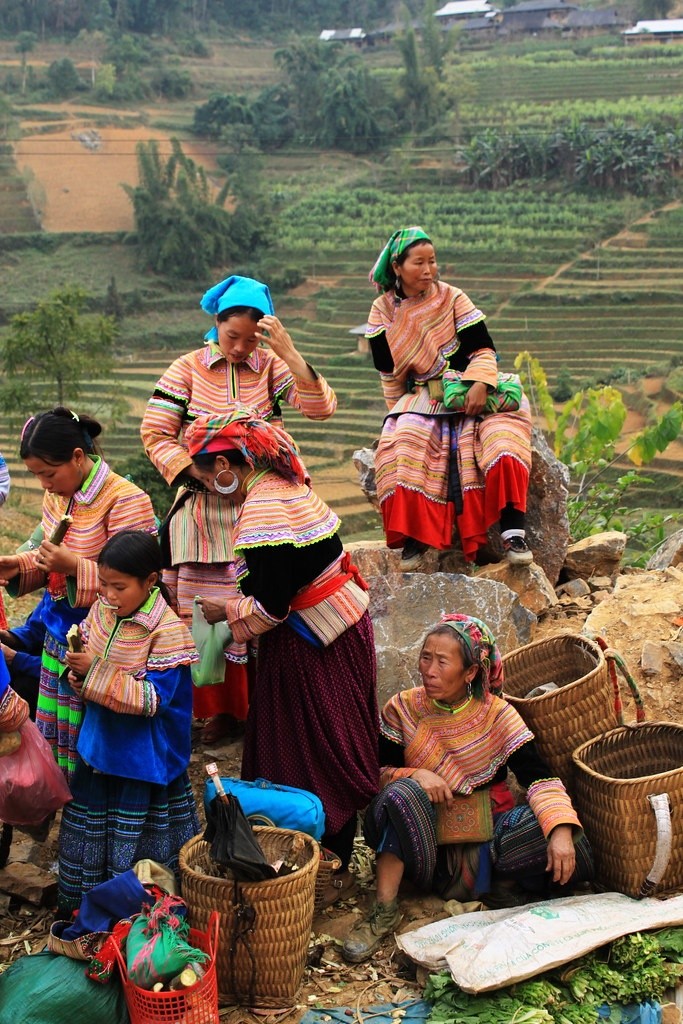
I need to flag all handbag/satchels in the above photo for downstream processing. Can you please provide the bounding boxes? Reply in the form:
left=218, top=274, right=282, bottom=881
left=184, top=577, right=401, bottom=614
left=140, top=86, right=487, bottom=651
left=0, top=715, right=74, bottom=825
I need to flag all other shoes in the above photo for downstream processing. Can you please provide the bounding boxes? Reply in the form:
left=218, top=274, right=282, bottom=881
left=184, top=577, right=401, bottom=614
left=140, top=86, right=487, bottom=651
left=399, top=539, right=424, bottom=572
left=341, top=899, right=403, bottom=963
left=504, top=536, right=533, bottom=566
left=200, top=718, right=219, bottom=743
left=324, top=872, right=358, bottom=908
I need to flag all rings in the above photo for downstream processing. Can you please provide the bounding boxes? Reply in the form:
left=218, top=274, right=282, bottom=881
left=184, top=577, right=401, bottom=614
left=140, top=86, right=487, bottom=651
left=41, top=557, right=45, bottom=561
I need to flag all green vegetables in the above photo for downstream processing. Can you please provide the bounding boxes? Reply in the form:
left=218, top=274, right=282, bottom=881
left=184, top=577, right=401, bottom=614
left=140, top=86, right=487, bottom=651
left=421, top=923, right=682, bottom=1024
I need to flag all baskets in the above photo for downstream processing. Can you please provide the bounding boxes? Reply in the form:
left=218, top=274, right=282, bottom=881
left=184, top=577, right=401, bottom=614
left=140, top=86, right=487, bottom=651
left=313, top=838, right=341, bottom=924
left=110, top=912, right=222, bottom=1024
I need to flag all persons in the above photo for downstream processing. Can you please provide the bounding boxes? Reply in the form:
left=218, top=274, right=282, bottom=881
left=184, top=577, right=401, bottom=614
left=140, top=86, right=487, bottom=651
left=140, top=275, right=337, bottom=743
left=363, top=226, right=534, bottom=572
left=0, top=406, right=158, bottom=787
left=343, top=613, right=584, bottom=963
left=186, top=408, right=381, bottom=909
left=56, top=530, right=200, bottom=910
left=0, top=600, right=46, bottom=870
